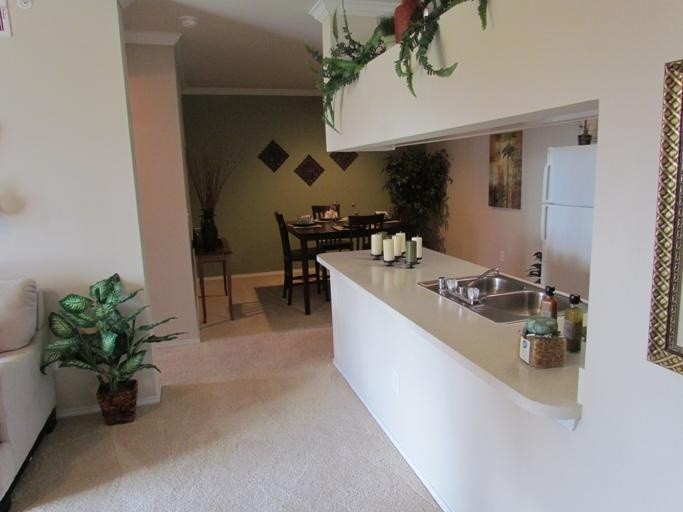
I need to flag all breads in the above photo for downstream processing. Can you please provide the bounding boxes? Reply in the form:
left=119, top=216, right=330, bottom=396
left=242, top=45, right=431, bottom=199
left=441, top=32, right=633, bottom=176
left=324, top=210, right=338, bottom=220
left=296, top=214, right=313, bottom=224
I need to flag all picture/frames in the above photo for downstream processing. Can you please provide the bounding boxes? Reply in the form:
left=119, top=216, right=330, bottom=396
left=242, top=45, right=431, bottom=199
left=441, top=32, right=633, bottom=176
left=647, top=59, right=683, bottom=374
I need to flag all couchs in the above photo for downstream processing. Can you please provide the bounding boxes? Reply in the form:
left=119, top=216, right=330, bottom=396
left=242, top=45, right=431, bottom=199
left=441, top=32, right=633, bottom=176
left=0, top=286, right=57, bottom=512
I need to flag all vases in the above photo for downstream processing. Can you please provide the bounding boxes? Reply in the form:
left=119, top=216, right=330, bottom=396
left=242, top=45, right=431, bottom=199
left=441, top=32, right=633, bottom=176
left=578, top=134, right=592, bottom=145
left=201, top=213, right=218, bottom=251
left=395, top=2, right=419, bottom=42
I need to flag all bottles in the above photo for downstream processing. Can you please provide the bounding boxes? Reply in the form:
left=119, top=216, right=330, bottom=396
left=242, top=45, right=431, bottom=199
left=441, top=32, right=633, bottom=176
left=538, top=285, right=583, bottom=352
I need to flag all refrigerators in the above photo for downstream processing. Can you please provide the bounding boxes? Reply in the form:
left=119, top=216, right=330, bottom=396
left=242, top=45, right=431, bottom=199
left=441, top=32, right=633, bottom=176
left=539, top=144, right=596, bottom=301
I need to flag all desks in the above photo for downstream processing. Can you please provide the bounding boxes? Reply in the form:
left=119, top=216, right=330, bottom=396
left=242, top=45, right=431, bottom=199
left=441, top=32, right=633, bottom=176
left=194, top=238, right=236, bottom=325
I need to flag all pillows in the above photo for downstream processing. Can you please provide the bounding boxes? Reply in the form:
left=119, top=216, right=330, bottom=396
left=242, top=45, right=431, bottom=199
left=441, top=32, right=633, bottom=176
left=1, top=277, right=37, bottom=354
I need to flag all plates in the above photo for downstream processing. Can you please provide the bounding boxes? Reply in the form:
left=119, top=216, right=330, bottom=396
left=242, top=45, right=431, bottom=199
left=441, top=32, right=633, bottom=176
left=293, top=221, right=317, bottom=226
left=320, top=217, right=340, bottom=221
left=343, top=223, right=367, bottom=229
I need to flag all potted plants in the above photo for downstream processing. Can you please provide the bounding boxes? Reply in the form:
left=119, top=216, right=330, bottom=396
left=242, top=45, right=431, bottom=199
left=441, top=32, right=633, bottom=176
left=40, top=274, right=190, bottom=425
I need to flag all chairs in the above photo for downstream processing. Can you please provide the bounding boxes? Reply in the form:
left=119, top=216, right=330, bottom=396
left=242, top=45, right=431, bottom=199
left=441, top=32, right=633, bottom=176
left=274, top=205, right=420, bottom=315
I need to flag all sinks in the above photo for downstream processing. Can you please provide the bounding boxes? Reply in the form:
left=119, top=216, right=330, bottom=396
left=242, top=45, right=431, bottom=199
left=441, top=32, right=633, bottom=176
left=462, top=291, right=588, bottom=323
left=416, top=273, right=538, bottom=301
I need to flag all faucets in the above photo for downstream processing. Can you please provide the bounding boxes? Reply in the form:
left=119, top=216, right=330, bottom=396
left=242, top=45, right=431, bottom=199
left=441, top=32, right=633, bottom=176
left=458, top=264, right=501, bottom=296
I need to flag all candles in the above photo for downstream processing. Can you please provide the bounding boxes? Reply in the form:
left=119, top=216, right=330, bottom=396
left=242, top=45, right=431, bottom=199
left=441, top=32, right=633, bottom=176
left=411, top=236, right=423, bottom=259
